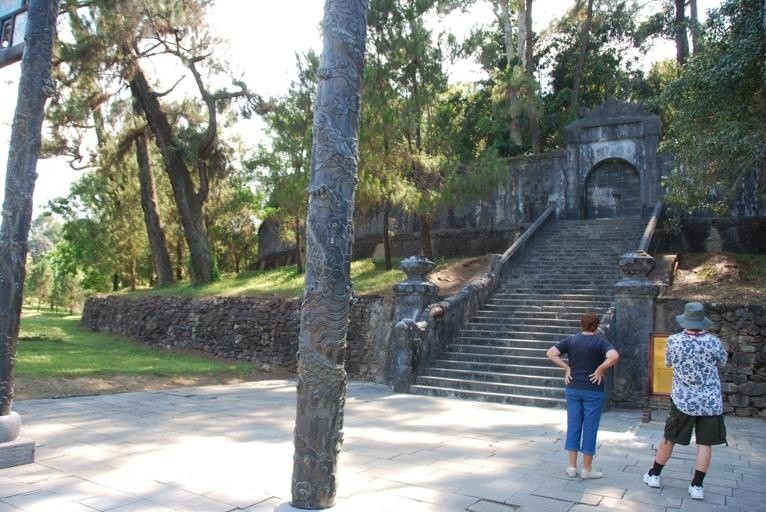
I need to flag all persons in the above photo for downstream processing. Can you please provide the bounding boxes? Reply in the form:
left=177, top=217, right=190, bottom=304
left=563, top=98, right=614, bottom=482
left=545, top=313, right=619, bottom=481
left=641, top=301, right=731, bottom=500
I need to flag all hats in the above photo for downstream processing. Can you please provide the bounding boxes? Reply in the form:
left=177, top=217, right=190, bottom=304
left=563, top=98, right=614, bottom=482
left=675, top=302, right=713, bottom=330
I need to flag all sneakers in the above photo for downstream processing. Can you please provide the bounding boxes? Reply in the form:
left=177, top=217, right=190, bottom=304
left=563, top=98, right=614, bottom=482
left=565, top=466, right=578, bottom=477
left=688, top=483, right=704, bottom=500
left=643, top=467, right=663, bottom=488
left=580, top=468, right=603, bottom=479
